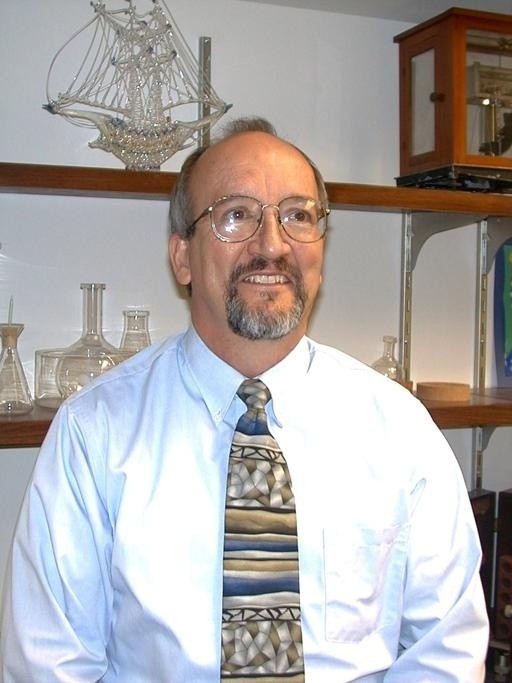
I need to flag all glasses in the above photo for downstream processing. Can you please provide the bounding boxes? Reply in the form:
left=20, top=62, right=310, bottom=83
left=184, top=194, right=333, bottom=243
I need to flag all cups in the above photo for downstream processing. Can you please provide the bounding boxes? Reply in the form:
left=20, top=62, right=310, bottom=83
left=35, top=350, right=79, bottom=411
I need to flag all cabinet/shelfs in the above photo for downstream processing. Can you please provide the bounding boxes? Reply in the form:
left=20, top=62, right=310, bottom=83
left=0, top=7, right=512, bottom=449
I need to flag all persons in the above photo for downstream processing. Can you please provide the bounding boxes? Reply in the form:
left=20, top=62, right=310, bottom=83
left=1, top=112, right=490, bottom=683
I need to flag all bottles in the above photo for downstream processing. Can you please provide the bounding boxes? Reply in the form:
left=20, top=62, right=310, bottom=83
left=1, top=297, right=33, bottom=418
left=55, top=282, right=127, bottom=400
left=371, top=336, right=403, bottom=386
left=116, top=308, right=152, bottom=362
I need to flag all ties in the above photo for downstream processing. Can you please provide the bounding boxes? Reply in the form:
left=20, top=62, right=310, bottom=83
left=218, top=378, right=309, bottom=683
